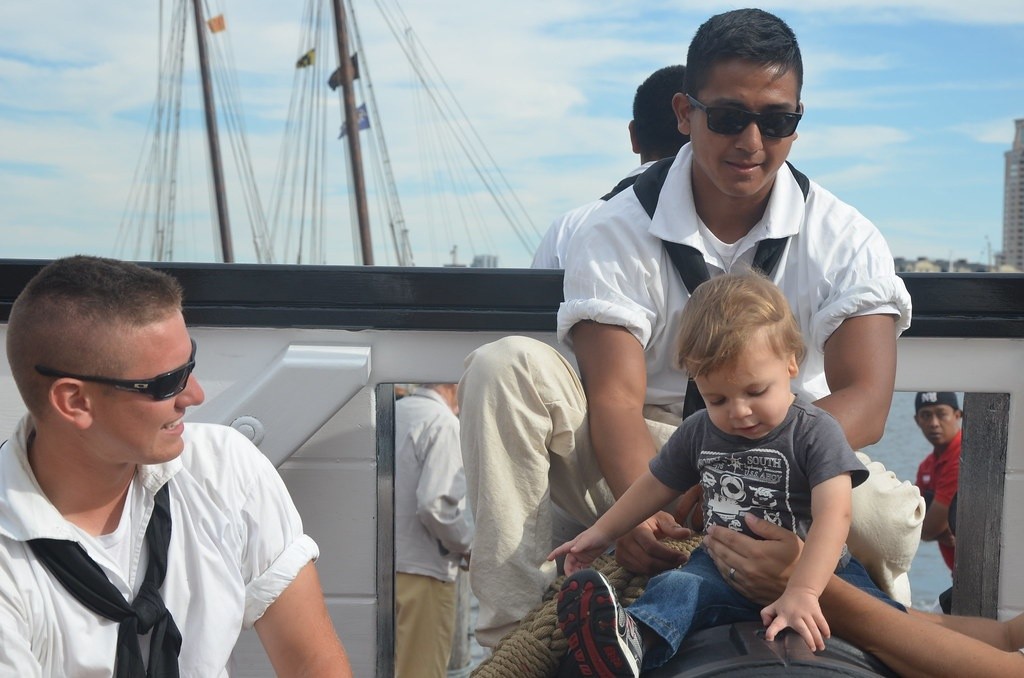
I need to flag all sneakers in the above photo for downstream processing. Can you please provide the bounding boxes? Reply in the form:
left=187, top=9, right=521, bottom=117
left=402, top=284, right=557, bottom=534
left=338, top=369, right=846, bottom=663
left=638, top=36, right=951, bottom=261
left=557, top=569, right=643, bottom=678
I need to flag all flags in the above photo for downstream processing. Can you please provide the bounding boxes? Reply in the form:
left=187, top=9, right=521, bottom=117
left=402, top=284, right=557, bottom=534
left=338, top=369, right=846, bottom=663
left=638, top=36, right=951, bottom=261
left=206, top=14, right=371, bottom=141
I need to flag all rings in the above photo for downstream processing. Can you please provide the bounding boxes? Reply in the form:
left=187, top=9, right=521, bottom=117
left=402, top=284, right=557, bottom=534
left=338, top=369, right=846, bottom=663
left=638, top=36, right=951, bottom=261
left=729, top=568, right=735, bottom=580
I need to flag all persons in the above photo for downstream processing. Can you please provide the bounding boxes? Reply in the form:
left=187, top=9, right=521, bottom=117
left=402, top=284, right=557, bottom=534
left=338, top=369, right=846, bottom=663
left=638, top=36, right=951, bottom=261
left=0, top=257, right=353, bottom=678
left=703, top=513, right=1024, bottom=678
left=394, top=384, right=473, bottom=678
left=459, top=9, right=927, bottom=650
left=914, top=392, right=963, bottom=614
left=547, top=274, right=870, bottom=678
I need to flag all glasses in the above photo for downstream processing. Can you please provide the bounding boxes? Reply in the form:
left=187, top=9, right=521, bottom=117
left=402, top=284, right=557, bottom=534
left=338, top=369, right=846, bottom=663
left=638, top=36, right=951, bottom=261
left=33, top=338, right=199, bottom=402
left=686, top=93, right=805, bottom=137
